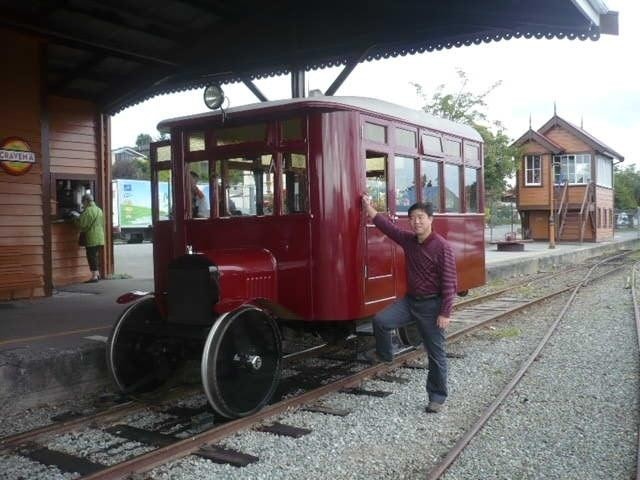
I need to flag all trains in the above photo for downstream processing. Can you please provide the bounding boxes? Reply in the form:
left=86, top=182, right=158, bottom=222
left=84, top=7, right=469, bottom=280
left=101, top=83, right=489, bottom=419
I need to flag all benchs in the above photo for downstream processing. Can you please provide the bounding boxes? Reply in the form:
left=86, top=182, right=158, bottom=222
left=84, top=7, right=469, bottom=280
left=486, top=235, right=538, bottom=253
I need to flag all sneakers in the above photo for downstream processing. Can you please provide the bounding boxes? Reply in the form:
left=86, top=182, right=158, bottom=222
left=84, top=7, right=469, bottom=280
left=83, top=274, right=101, bottom=283
left=425, top=401, right=446, bottom=413
left=364, top=350, right=394, bottom=366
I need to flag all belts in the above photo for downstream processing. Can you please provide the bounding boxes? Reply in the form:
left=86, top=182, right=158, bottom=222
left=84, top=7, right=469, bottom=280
left=406, top=293, right=440, bottom=301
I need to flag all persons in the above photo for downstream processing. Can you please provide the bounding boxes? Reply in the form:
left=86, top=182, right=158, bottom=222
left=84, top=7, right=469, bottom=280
left=359, top=192, right=458, bottom=413
left=62, top=192, right=107, bottom=283
left=217, top=177, right=238, bottom=218
left=190, top=170, right=207, bottom=218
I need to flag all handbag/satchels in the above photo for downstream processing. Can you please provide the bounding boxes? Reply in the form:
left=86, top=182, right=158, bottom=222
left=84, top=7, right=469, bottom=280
left=78, top=232, right=85, bottom=246
